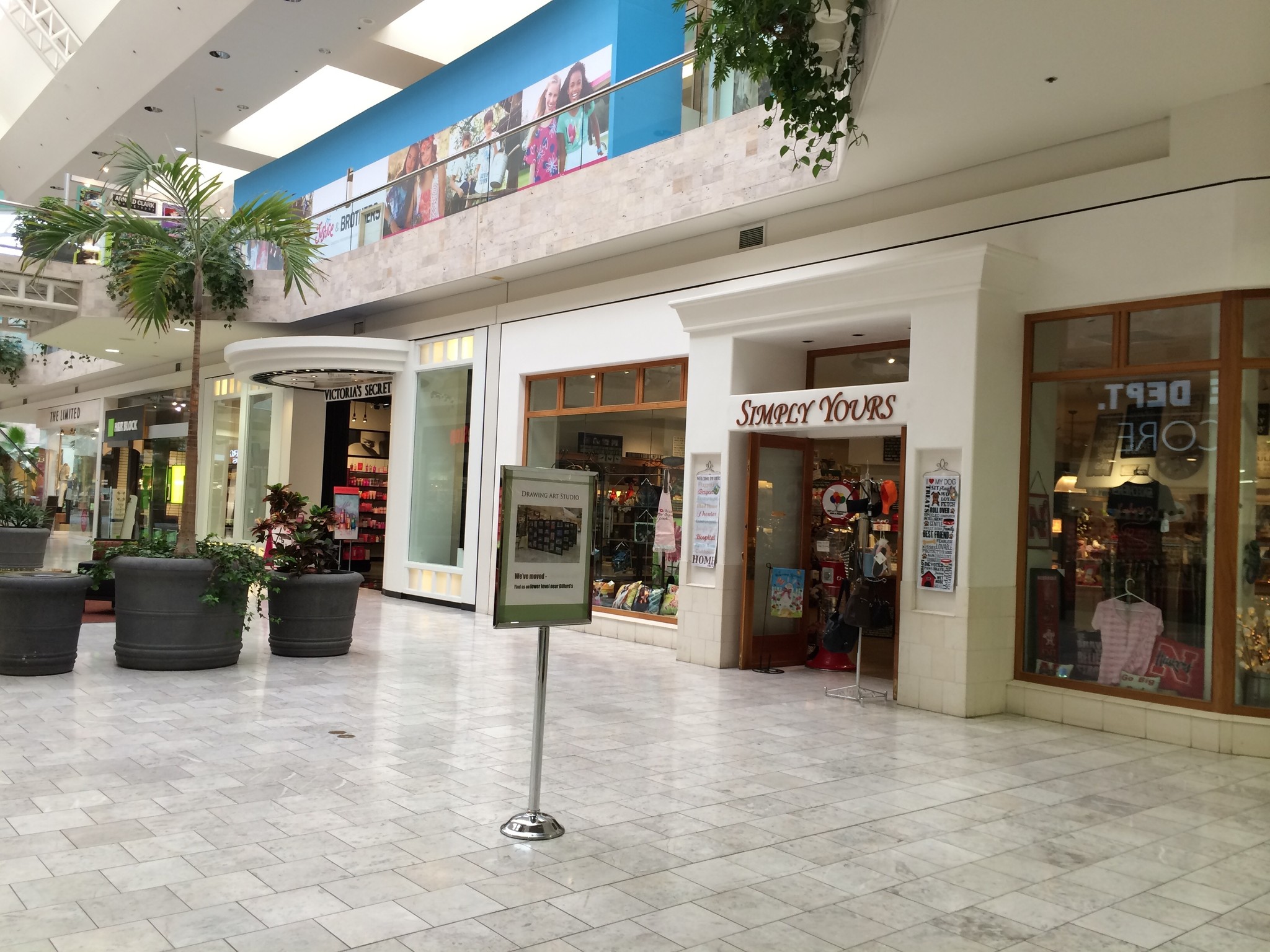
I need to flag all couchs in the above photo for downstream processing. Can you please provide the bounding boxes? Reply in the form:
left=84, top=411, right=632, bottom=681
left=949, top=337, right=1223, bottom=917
left=78, top=537, right=142, bottom=613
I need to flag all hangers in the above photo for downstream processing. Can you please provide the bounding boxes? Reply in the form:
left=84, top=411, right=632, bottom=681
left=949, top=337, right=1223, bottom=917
left=1127, top=465, right=1156, bottom=482
left=1113, top=578, right=1148, bottom=604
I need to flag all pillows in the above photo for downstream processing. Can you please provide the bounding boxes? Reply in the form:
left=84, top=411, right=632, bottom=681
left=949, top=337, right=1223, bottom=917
left=630, top=584, right=665, bottom=615
left=591, top=578, right=615, bottom=608
left=611, top=580, right=643, bottom=611
left=660, top=583, right=679, bottom=615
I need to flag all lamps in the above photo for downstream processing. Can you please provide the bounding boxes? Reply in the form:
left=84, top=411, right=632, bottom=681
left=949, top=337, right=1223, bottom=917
left=1051, top=518, right=1063, bottom=533
left=362, top=402, right=367, bottom=423
left=373, top=403, right=380, bottom=409
left=1053, top=409, right=1087, bottom=493
left=382, top=403, right=390, bottom=410
left=352, top=401, right=357, bottom=422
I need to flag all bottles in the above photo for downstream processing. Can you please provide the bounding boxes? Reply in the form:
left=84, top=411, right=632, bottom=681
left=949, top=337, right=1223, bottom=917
left=366, top=464, right=376, bottom=472
left=340, top=509, right=356, bottom=529
left=350, top=463, right=357, bottom=471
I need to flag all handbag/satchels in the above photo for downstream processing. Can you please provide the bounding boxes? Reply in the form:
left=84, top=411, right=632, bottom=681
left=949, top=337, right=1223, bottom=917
left=822, top=476, right=898, bottom=654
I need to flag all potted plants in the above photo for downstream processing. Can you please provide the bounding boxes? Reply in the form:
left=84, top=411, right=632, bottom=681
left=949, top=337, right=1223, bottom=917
left=76, top=526, right=299, bottom=672
left=665, top=0, right=869, bottom=180
left=0, top=467, right=57, bottom=570
left=246, top=483, right=365, bottom=657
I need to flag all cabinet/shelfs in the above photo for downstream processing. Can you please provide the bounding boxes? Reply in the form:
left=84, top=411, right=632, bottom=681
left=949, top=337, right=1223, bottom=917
left=555, top=451, right=685, bottom=578
left=346, top=467, right=389, bottom=562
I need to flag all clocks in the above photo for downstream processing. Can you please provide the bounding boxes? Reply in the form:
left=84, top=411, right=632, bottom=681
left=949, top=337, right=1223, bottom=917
left=1155, top=434, right=1205, bottom=480
